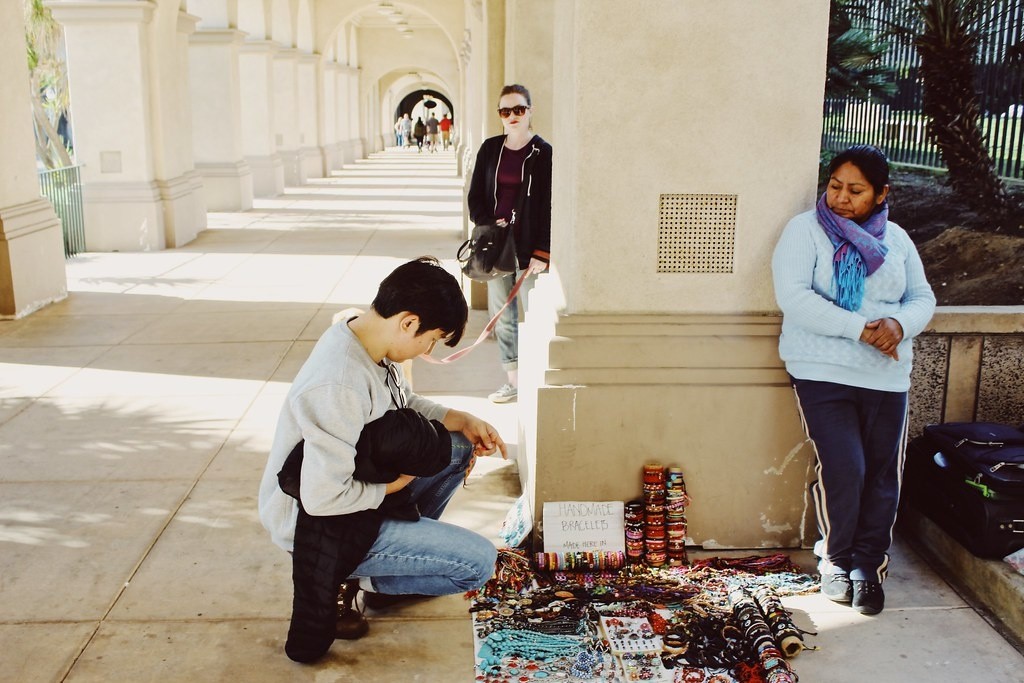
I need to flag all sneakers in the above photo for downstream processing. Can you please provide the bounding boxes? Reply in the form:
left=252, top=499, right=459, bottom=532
left=852, top=580, right=884, bottom=615
left=821, top=573, right=853, bottom=601
left=488, top=383, right=517, bottom=403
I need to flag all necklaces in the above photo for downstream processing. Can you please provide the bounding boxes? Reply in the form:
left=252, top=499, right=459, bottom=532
left=495, top=548, right=535, bottom=592
left=463, top=574, right=608, bottom=660
left=589, top=553, right=821, bottom=617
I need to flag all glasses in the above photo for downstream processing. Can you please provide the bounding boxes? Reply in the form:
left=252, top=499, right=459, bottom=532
left=383, top=362, right=407, bottom=409
left=498, top=105, right=529, bottom=119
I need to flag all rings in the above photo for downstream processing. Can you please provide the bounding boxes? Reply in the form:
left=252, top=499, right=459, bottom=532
left=623, top=653, right=662, bottom=682
left=606, top=619, right=657, bottom=651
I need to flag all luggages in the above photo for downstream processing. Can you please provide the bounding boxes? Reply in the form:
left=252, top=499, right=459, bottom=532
left=903, top=422, right=1024, bottom=560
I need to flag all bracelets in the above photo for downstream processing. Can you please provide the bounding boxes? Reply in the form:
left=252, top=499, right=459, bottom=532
left=624, top=464, right=692, bottom=565
left=463, top=440, right=483, bottom=491
left=661, top=584, right=821, bottom=683
left=476, top=651, right=625, bottom=683
left=535, top=550, right=625, bottom=573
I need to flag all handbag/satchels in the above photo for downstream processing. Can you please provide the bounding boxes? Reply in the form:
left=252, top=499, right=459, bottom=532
left=456, top=222, right=515, bottom=284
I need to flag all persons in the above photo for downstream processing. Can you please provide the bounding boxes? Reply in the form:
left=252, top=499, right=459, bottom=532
left=426, top=112, right=440, bottom=153
left=440, top=113, right=451, bottom=151
left=414, top=117, right=426, bottom=153
left=468, top=84, right=552, bottom=403
left=771, top=145, right=937, bottom=617
left=258, top=257, right=509, bottom=639
left=395, top=113, right=411, bottom=146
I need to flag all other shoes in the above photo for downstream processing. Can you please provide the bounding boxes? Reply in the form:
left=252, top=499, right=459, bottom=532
left=334, top=578, right=368, bottom=639
left=362, top=591, right=428, bottom=610
